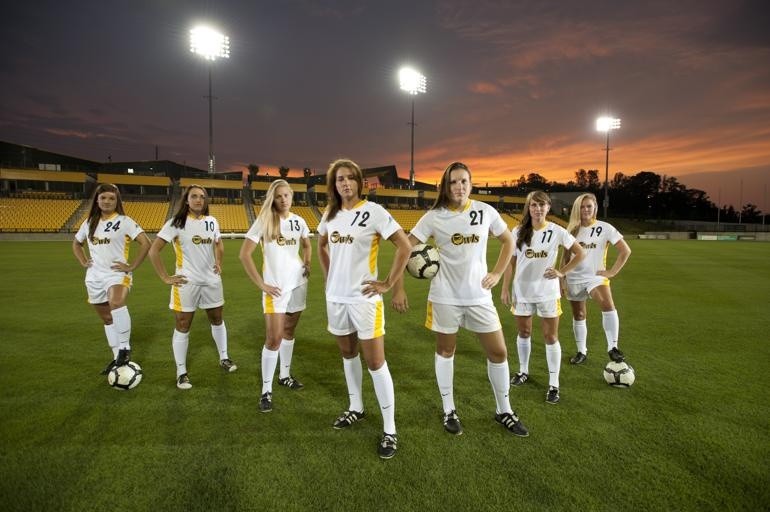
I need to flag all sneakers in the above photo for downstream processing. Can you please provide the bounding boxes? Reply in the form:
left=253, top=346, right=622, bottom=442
left=177, top=373, right=192, bottom=389
left=570, top=351, right=586, bottom=364
left=378, top=432, right=398, bottom=460
left=220, top=359, right=237, bottom=372
left=495, top=411, right=529, bottom=437
left=608, top=347, right=625, bottom=362
left=260, top=392, right=272, bottom=412
left=99, top=360, right=115, bottom=375
left=442, top=410, right=463, bottom=436
left=544, top=387, right=560, bottom=404
left=278, top=375, right=305, bottom=391
left=331, top=409, right=365, bottom=430
left=510, top=372, right=529, bottom=387
left=115, top=349, right=130, bottom=366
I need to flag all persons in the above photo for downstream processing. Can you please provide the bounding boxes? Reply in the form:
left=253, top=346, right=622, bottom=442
left=390, top=163, right=531, bottom=438
left=317, top=160, right=411, bottom=460
left=561, top=193, right=632, bottom=365
left=238, top=179, right=312, bottom=412
left=501, top=191, right=584, bottom=406
left=71, top=184, right=151, bottom=378
left=148, top=184, right=237, bottom=388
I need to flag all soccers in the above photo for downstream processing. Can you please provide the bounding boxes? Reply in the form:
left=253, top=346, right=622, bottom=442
left=405, top=242, right=441, bottom=281
left=108, top=360, right=143, bottom=393
left=603, top=360, right=636, bottom=388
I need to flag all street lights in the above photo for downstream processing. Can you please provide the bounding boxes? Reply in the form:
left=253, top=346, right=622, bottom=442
left=188, top=25, right=230, bottom=205
left=190, top=27, right=229, bottom=178
left=397, top=64, right=425, bottom=186
left=400, top=68, right=426, bottom=190
